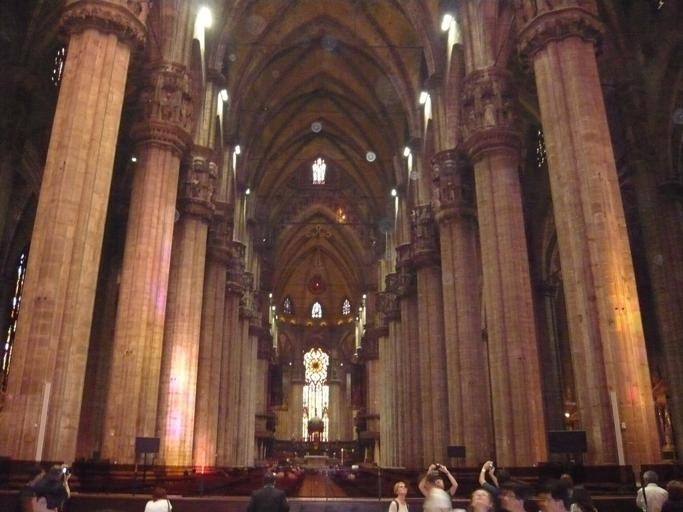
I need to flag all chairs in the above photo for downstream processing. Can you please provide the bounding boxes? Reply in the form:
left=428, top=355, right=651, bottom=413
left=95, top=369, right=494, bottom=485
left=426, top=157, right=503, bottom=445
left=158, top=465, right=419, bottom=500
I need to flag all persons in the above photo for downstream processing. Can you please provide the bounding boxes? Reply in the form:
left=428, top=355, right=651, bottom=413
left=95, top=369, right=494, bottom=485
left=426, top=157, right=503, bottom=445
left=17, top=466, right=45, bottom=511
left=635, top=469, right=668, bottom=511
left=144, top=486, right=171, bottom=511
left=34, top=463, right=70, bottom=511
left=247, top=472, right=289, bottom=511
left=388, top=460, right=597, bottom=511
left=661, top=480, right=683, bottom=512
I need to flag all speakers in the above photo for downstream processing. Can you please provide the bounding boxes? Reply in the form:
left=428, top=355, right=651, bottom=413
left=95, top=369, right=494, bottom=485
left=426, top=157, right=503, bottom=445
left=448, top=446, right=465, bottom=457
left=136, top=437, right=160, bottom=453
left=549, top=431, right=586, bottom=453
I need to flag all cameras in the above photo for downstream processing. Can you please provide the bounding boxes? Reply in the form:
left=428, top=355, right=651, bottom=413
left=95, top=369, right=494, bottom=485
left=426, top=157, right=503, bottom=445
left=434, top=464, right=441, bottom=470
left=489, top=461, right=494, bottom=469
